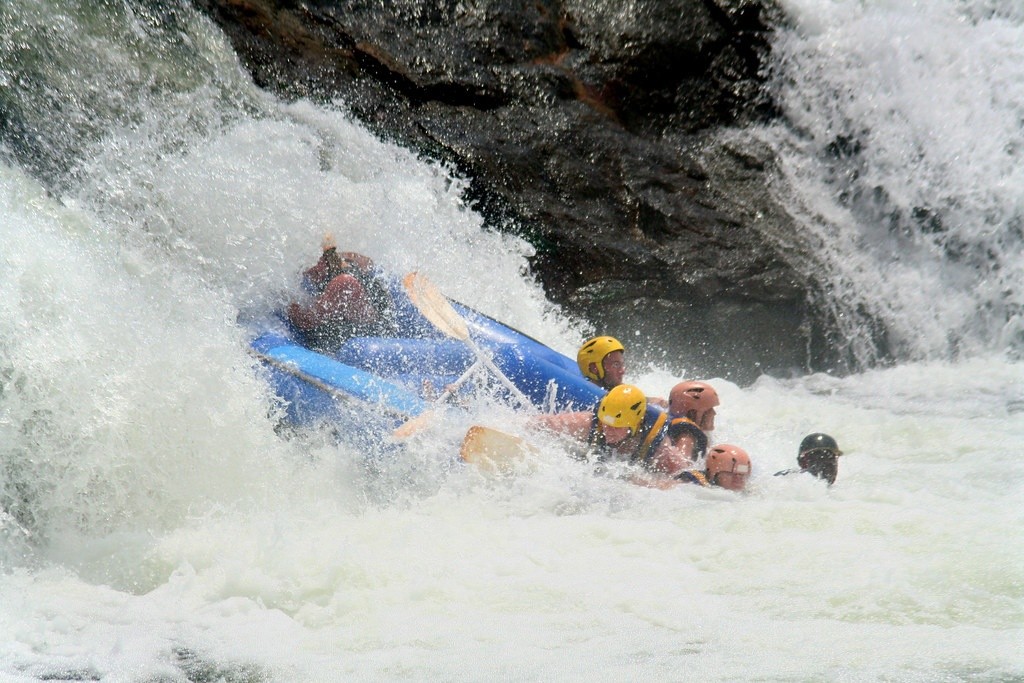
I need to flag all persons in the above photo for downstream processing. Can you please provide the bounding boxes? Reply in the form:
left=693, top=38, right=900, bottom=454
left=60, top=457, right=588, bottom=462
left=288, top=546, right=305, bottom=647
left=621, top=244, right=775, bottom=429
left=290, top=248, right=399, bottom=353
left=534, top=335, right=844, bottom=494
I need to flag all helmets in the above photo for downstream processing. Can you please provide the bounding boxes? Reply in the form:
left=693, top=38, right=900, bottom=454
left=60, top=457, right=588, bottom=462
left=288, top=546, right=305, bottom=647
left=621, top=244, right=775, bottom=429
left=669, top=381, right=721, bottom=426
left=577, top=336, right=624, bottom=381
left=797, top=432, right=844, bottom=468
left=597, top=384, right=647, bottom=437
left=705, top=444, right=752, bottom=484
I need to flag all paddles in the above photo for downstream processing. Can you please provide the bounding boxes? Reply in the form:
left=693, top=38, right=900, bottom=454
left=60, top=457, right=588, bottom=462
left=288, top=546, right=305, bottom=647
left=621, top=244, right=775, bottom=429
left=405, top=268, right=546, bottom=413
left=392, top=354, right=490, bottom=443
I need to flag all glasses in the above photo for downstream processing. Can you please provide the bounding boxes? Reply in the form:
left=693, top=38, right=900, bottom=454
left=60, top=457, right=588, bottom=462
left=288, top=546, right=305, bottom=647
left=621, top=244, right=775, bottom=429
left=602, top=361, right=625, bottom=369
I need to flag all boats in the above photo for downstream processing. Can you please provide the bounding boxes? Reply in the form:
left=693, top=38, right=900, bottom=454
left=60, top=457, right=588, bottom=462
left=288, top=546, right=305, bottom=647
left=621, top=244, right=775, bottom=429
left=236, top=266, right=754, bottom=507
